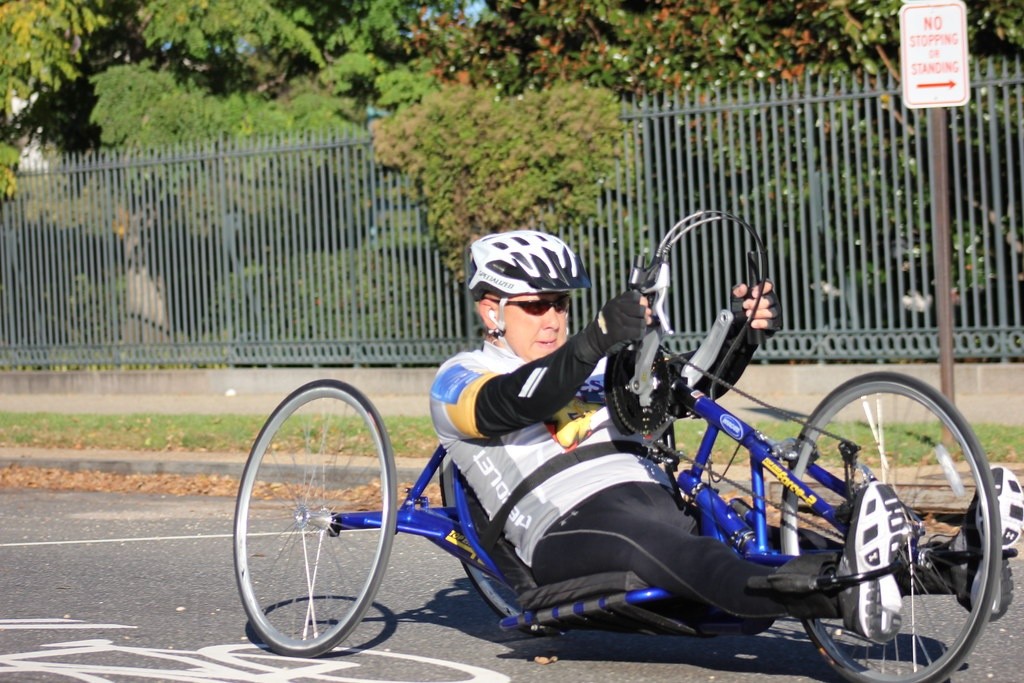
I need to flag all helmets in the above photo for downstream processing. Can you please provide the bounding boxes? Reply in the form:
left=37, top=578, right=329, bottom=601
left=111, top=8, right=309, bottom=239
left=468, top=230, right=593, bottom=302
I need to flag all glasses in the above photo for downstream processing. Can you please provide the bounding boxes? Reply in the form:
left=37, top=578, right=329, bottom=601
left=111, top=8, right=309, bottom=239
left=483, top=295, right=570, bottom=316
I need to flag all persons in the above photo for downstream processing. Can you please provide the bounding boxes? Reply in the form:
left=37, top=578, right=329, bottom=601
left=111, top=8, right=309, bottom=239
left=429, top=229, right=1024, bottom=645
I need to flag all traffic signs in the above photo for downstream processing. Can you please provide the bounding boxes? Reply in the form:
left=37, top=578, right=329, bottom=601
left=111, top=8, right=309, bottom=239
left=899, top=2, right=971, bottom=107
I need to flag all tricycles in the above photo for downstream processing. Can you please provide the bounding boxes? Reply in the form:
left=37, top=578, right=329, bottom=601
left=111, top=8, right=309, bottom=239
left=233, top=211, right=1018, bottom=683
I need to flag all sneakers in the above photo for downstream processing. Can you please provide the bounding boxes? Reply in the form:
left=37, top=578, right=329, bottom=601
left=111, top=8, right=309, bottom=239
left=957, top=465, right=1024, bottom=623
left=837, top=481, right=905, bottom=643
